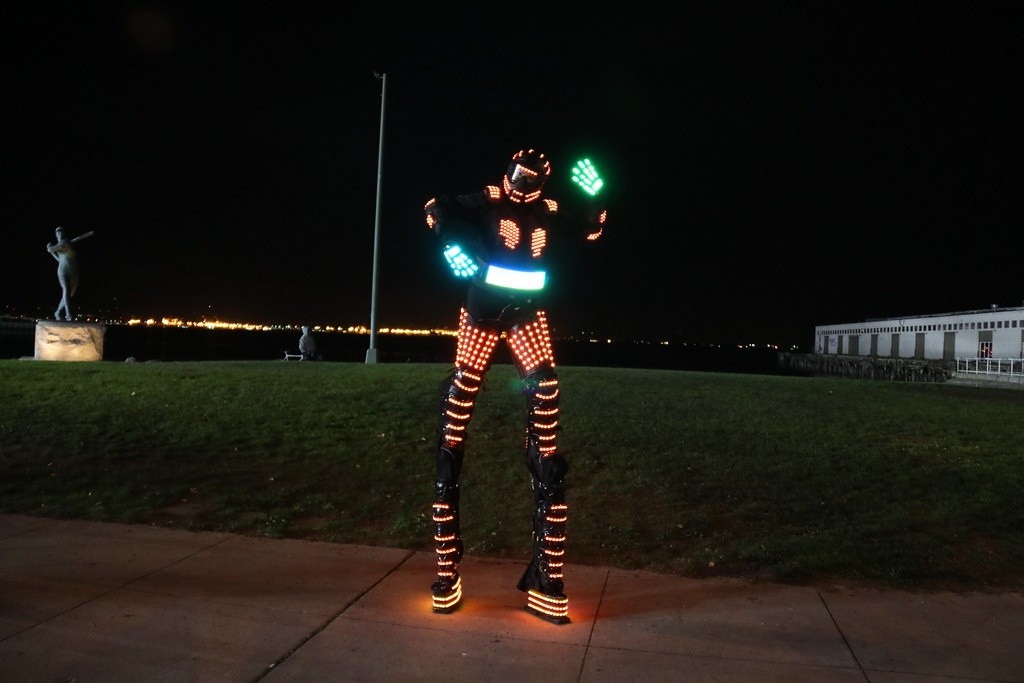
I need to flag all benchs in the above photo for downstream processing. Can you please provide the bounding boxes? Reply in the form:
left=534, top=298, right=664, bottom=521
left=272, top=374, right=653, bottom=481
left=284, top=351, right=312, bottom=362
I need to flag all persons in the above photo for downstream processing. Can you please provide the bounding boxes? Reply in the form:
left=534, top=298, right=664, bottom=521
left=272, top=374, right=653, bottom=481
left=47, top=228, right=79, bottom=321
left=422, top=150, right=607, bottom=623
left=298, top=326, right=316, bottom=360
